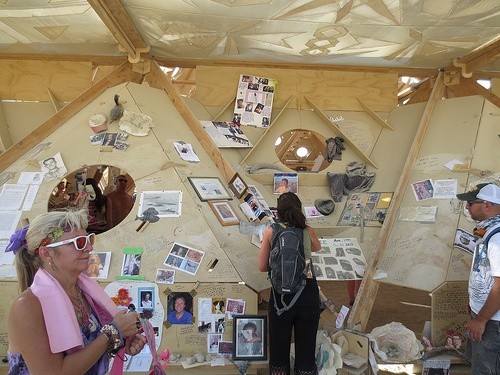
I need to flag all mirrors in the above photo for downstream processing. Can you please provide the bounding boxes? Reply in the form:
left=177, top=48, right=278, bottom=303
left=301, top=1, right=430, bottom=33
left=47, top=164, right=137, bottom=235
left=274, top=129, right=333, bottom=174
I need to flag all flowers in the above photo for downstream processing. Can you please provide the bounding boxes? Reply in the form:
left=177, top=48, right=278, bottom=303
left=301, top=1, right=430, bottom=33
left=53, top=228, right=64, bottom=237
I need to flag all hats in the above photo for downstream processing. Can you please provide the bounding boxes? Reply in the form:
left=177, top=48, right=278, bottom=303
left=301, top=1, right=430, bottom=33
left=456, top=183, right=500, bottom=205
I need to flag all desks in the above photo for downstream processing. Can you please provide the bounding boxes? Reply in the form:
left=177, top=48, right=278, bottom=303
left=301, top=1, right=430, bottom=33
left=152, top=354, right=465, bottom=375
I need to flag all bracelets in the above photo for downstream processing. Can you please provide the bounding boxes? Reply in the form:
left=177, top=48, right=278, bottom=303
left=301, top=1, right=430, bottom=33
left=101, top=324, right=119, bottom=357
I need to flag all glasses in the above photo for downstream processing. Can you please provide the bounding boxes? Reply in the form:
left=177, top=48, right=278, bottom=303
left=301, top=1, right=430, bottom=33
left=117, top=179, right=128, bottom=183
left=46, top=233, right=95, bottom=250
left=467, top=200, right=489, bottom=206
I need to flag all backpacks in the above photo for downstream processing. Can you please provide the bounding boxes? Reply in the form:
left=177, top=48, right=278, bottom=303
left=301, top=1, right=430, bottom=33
left=268, top=223, right=310, bottom=294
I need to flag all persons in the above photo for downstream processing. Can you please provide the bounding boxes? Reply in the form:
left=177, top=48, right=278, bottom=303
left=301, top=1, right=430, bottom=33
left=170, top=247, right=183, bottom=268
left=457, top=183, right=500, bottom=375
left=167, top=296, right=192, bottom=324
left=258, top=192, right=322, bottom=375
left=238, top=322, right=261, bottom=355
left=4, top=210, right=147, bottom=375
left=237, top=99, right=244, bottom=108
left=44, top=158, right=66, bottom=181
left=262, top=118, right=269, bottom=126
left=246, top=103, right=251, bottom=111
left=256, top=105, right=262, bottom=113
left=55, top=169, right=134, bottom=230
left=103, top=134, right=115, bottom=146
left=276, top=178, right=292, bottom=193
left=142, top=293, right=152, bottom=308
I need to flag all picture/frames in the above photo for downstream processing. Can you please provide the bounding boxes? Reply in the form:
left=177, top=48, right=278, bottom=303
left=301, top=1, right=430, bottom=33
left=187, top=177, right=233, bottom=202
left=233, top=315, right=267, bottom=361
left=229, top=172, right=248, bottom=200
left=207, top=200, right=241, bottom=226
